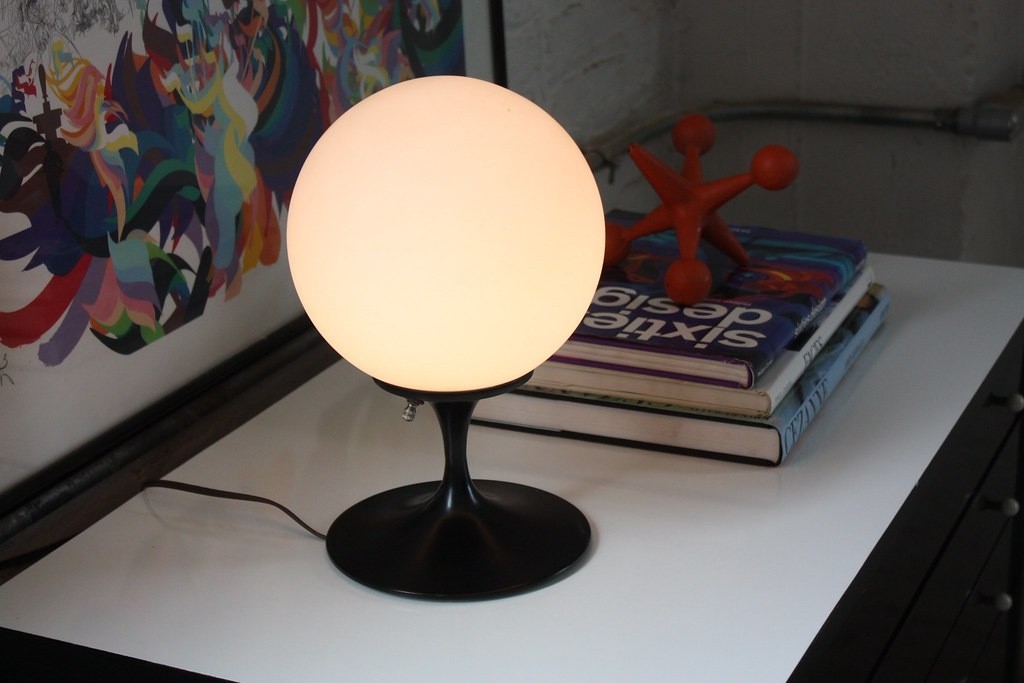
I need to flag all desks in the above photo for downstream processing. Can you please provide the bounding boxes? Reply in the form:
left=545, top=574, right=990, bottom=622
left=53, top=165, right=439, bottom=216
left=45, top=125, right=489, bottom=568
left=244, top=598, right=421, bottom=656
left=0, top=201, right=1024, bottom=682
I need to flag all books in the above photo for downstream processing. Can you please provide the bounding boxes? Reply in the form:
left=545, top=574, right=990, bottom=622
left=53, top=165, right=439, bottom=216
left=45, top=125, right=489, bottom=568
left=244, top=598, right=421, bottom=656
left=471, top=208, right=891, bottom=467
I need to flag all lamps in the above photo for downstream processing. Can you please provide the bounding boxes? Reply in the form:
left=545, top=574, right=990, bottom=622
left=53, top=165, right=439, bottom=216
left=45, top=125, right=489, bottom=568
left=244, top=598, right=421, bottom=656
left=284, top=75, right=606, bottom=602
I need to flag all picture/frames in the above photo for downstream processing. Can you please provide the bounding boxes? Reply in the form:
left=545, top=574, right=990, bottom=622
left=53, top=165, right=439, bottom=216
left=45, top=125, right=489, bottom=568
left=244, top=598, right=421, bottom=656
left=0, top=0, right=507, bottom=518
